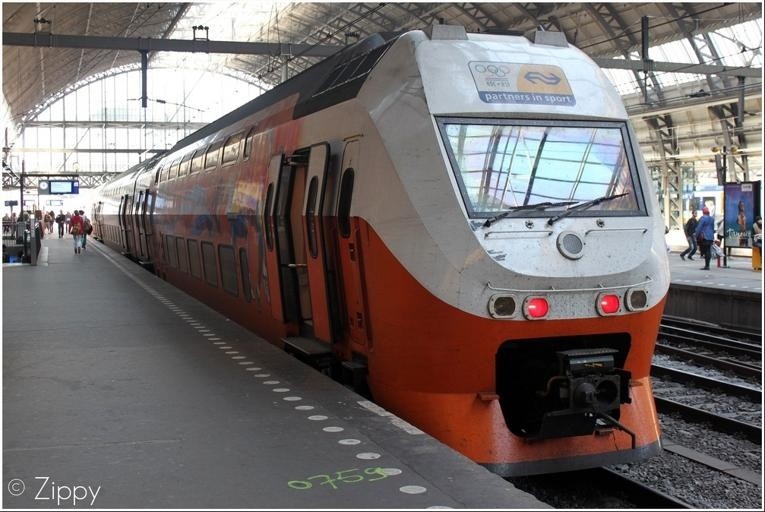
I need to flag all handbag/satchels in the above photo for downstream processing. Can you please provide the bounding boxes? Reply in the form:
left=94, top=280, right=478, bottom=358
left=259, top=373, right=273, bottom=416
left=710, top=244, right=725, bottom=260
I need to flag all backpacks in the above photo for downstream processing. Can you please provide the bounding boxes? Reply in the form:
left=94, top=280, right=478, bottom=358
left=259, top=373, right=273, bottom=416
left=70, top=222, right=82, bottom=234
left=85, top=224, right=93, bottom=235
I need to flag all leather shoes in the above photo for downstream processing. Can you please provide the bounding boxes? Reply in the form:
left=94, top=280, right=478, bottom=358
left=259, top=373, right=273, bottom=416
left=680, top=254, right=694, bottom=261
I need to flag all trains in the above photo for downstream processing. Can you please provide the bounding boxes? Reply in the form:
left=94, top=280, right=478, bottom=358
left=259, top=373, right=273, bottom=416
left=91, top=22, right=665, bottom=483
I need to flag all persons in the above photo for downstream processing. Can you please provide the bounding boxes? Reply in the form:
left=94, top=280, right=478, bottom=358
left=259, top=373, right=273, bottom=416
left=2, top=208, right=92, bottom=263
left=716, top=218, right=725, bottom=248
left=679, top=208, right=699, bottom=262
left=695, top=206, right=714, bottom=270
left=751, top=215, right=762, bottom=250
left=737, top=200, right=747, bottom=234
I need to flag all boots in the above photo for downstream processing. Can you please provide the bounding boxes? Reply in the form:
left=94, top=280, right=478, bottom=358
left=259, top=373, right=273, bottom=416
left=74, top=247, right=81, bottom=254
